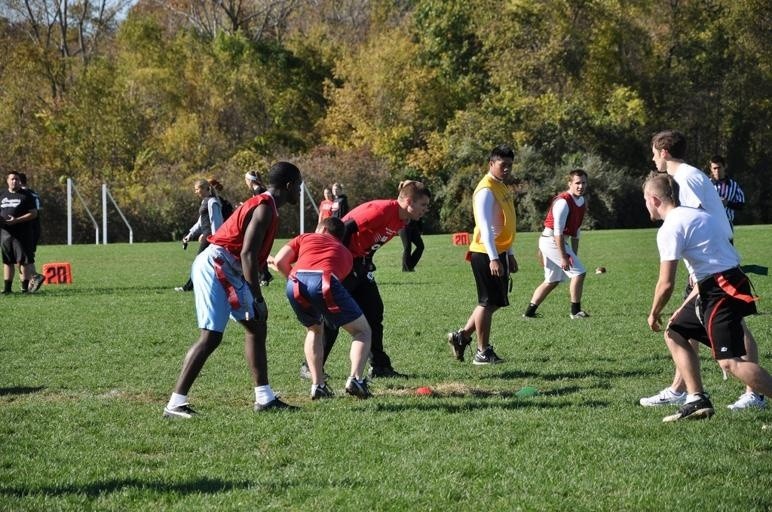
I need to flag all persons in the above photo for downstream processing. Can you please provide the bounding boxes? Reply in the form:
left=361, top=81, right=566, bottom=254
left=446, top=144, right=519, bottom=367
left=636, top=127, right=770, bottom=413
left=313, top=184, right=334, bottom=223
left=704, top=154, right=747, bottom=245
left=523, top=169, right=593, bottom=319
left=163, top=160, right=304, bottom=420
left=0, top=169, right=39, bottom=295
left=298, top=177, right=434, bottom=381
left=639, top=171, right=771, bottom=426
left=329, top=181, right=350, bottom=218
left=15, top=171, right=46, bottom=294
left=173, top=178, right=226, bottom=293
left=243, top=169, right=275, bottom=288
left=273, top=215, right=375, bottom=400
left=206, top=176, right=234, bottom=222
left=397, top=177, right=425, bottom=275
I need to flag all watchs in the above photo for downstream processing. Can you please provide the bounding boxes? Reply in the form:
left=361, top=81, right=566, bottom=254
left=254, top=294, right=266, bottom=306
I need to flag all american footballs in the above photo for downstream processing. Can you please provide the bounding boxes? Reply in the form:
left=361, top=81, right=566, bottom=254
left=595, top=266, right=606, bottom=275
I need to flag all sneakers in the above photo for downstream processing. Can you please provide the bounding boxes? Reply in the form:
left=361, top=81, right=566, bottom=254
left=639, top=386, right=687, bottom=407
left=173, top=286, right=187, bottom=293
left=661, top=391, right=715, bottom=426
left=1, top=289, right=13, bottom=296
left=447, top=328, right=474, bottom=362
left=29, top=272, right=46, bottom=294
left=20, top=287, right=29, bottom=296
left=472, top=343, right=504, bottom=366
left=344, top=376, right=374, bottom=400
left=161, top=404, right=199, bottom=419
left=725, top=392, right=769, bottom=411
left=253, top=396, right=302, bottom=414
left=309, top=381, right=337, bottom=401
left=568, top=310, right=590, bottom=321
left=300, top=362, right=331, bottom=380
left=371, top=366, right=409, bottom=381
left=266, top=275, right=274, bottom=284
left=259, top=281, right=268, bottom=289
left=521, top=313, right=543, bottom=321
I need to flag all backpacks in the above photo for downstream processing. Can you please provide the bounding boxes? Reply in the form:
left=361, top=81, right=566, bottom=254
left=218, top=196, right=234, bottom=221
